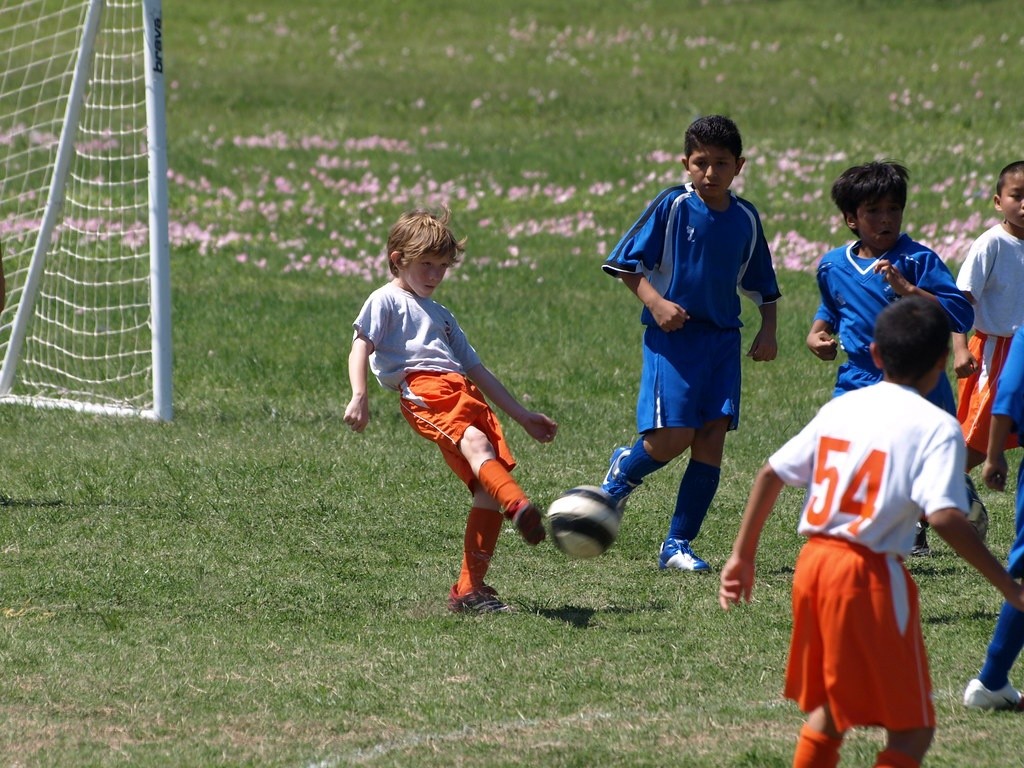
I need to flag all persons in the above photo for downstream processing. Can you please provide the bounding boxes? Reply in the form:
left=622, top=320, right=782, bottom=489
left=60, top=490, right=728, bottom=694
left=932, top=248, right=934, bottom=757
left=718, top=297, right=1023, bottom=768
left=343, top=211, right=560, bottom=613
left=952, top=159, right=1024, bottom=473
left=963, top=325, right=1023, bottom=709
left=600, top=116, right=782, bottom=571
left=805, top=161, right=989, bottom=556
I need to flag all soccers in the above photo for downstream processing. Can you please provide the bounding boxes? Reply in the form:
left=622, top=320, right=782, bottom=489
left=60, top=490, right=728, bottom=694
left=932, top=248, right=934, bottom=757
left=547, top=484, right=621, bottom=558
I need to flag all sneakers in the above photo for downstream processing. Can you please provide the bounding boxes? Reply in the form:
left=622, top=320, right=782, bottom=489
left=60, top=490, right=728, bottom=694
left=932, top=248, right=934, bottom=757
left=448, top=582, right=509, bottom=613
left=910, top=519, right=930, bottom=555
left=963, top=679, right=1024, bottom=710
left=600, top=446, right=644, bottom=511
left=658, top=537, right=711, bottom=571
left=503, top=499, right=546, bottom=545
